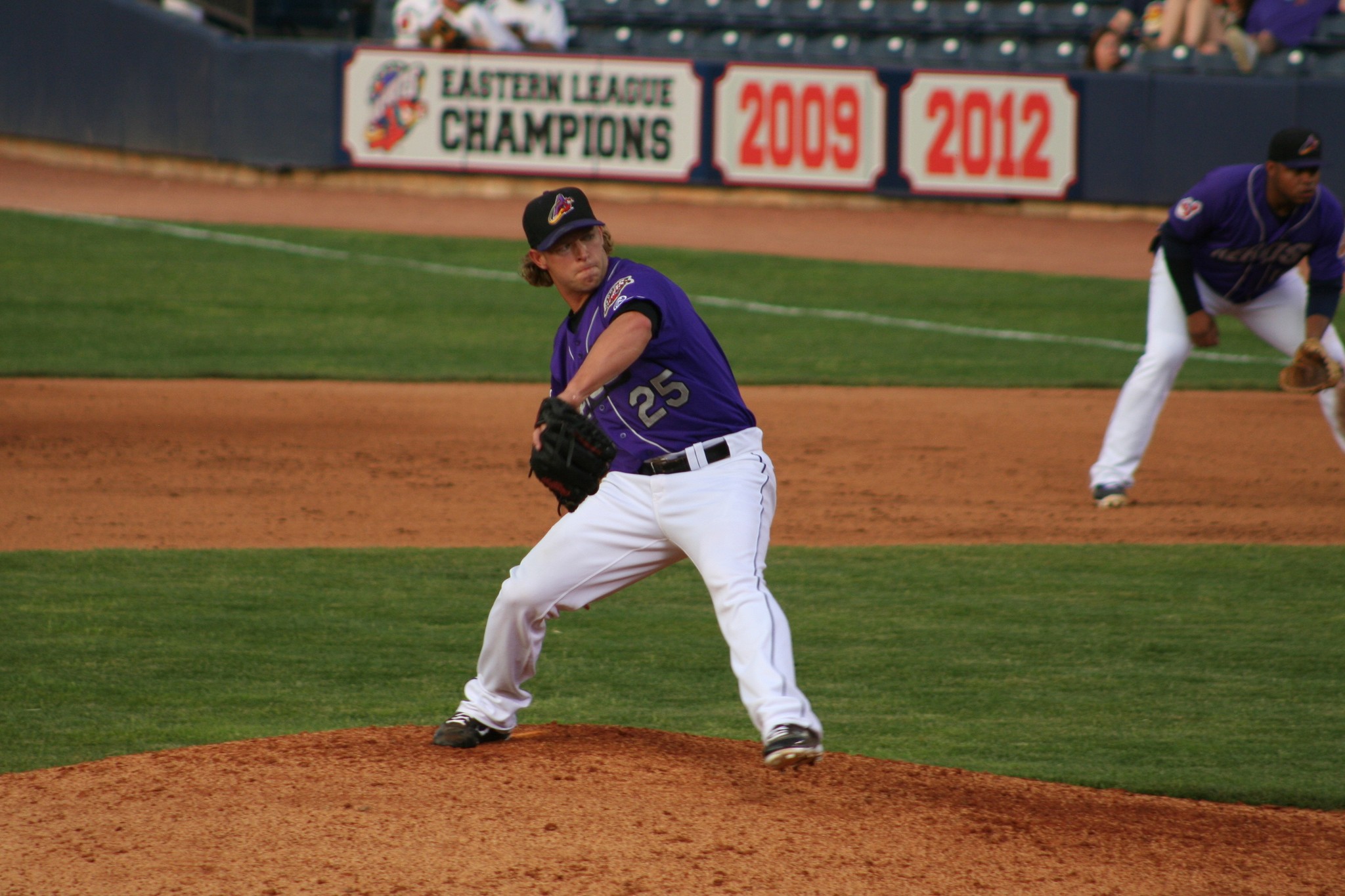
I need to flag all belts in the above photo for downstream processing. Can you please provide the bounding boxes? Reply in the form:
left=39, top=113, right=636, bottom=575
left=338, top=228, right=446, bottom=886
left=639, top=440, right=730, bottom=476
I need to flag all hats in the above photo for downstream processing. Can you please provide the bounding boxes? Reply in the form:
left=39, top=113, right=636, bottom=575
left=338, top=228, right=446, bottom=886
left=1267, top=123, right=1327, bottom=169
left=519, top=185, right=606, bottom=252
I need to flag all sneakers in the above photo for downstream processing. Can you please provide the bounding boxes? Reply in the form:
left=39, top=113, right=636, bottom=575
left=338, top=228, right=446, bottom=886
left=433, top=711, right=510, bottom=749
left=1093, top=482, right=1130, bottom=506
left=759, top=723, right=824, bottom=770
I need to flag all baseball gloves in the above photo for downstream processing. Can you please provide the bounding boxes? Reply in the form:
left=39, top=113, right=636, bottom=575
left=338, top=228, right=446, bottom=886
left=530, top=397, right=617, bottom=513
left=1279, top=337, right=1341, bottom=393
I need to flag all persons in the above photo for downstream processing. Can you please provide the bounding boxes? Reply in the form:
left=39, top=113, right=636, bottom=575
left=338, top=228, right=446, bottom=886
left=431, top=186, right=825, bottom=771
left=393, top=0, right=568, bottom=55
left=1086, top=122, right=1345, bottom=509
left=1089, top=0, right=1345, bottom=80
